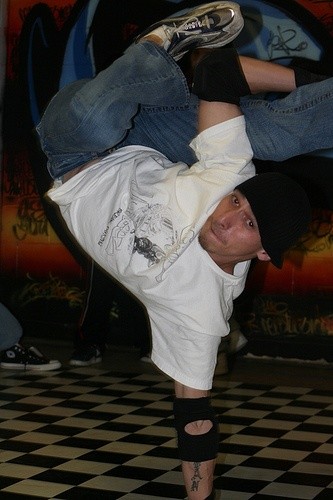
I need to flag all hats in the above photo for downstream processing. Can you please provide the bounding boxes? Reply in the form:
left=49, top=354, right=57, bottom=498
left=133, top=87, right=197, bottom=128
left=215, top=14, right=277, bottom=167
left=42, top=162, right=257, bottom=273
left=231, top=172, right=313, bottom=270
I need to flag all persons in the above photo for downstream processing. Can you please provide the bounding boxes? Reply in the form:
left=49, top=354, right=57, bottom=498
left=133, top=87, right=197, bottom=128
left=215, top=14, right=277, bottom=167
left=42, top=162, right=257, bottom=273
left=70, top=234, right=154, bottom=367
left=214, top=313, right=251, bottom=378
left=35, top=0, right=332, bottom=499
left=0, top=301, right=62, bottom=372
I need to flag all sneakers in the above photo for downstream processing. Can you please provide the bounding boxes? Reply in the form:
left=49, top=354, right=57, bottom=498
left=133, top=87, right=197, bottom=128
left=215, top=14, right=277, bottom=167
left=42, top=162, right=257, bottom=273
left=136, top=0, right=245, bottom=65
left=69, top=344, right=104, bottom=365
left=0, top=345, right=62, bottom=371
